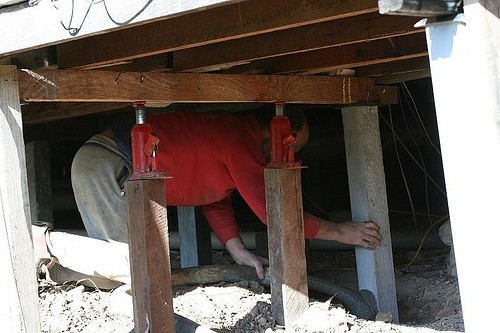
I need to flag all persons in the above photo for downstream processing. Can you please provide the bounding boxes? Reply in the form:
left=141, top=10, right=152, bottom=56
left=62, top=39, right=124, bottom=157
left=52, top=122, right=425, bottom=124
left=31, top=103, right=381, bottom=276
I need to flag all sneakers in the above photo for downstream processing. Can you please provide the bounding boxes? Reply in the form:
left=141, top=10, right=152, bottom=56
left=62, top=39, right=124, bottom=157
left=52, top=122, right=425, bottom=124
left=31, top=219, right=53, bottom=299
